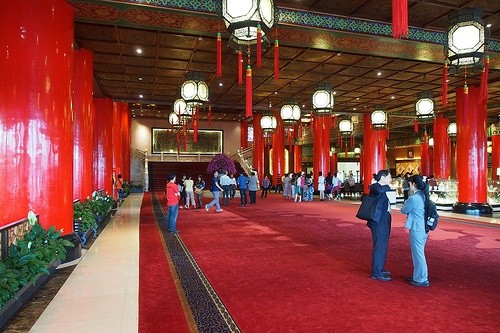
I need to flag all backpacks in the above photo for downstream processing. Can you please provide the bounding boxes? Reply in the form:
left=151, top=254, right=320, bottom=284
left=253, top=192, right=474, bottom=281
left=425, top=200, right=438, bottom=233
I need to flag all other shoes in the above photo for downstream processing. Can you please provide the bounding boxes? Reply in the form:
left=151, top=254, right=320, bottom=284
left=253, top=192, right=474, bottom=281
left=167, top=229, right=170, bottom=233
left=406, top=277, right=414, bottom=281
left=205, top=204, right=209, bottom=212
left=298, top=201, right=302, bottom=202
left=410, top=282, right=429, bottom=287
left=216, top=209, right=222, bottom=212
left=293, top=200, right=297, bottom=203
left=170, top=231, right=180, bottom=234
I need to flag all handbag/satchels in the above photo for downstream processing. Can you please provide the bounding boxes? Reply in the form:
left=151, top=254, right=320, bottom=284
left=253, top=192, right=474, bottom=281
left=356, top=193, right=384, bottom=223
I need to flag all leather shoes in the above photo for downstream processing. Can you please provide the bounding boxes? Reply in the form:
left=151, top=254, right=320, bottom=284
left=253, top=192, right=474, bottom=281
left=372, top=275, right=391, bottom=281
left=383, top=272, right=390, bottom=275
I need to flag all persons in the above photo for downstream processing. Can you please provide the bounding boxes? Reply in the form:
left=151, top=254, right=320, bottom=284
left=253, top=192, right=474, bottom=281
left=370, top=172, right=437, bottom=201
left=165, top=170, right=258, bottom=233
left=274, top=170, right=356, bottom=203
left=400, top=175, right=430, bottom=286
left=204, top=170, right=223, bottom=212
left=114, top=174, right=124, bottom=201
left=366, top=170, right=392, bottom=280
left=260, top=175, right=271, bottom=198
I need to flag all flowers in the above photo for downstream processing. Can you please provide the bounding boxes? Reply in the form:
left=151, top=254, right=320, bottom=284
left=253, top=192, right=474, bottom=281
left=74, top=190, right=116, bottom=230
left=0, top=210, right=75, bottom=308
left=206, top=154, right=237, bottom=174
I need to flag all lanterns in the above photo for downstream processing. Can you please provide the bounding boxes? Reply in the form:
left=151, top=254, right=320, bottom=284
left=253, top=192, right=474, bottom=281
left=337, top=90, right=436, bottom=154
left=441, top=7, right=489, bottom=125
left=168, top=72, right=211, bottom=153
left=259, top=82, right=336, bottom=150
left=216, top=0, right=279, bottom=118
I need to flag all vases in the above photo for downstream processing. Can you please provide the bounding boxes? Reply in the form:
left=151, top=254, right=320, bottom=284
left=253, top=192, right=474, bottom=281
left=218, top=169, right=227, bottom=180
left=0, top=254, right=63, bottom=329
left=85, top=225, right=96, bottom=240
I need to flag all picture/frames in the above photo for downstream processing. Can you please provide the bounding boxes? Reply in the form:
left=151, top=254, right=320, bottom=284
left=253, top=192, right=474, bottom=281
left=151, top=127, right=224, bottom=155
